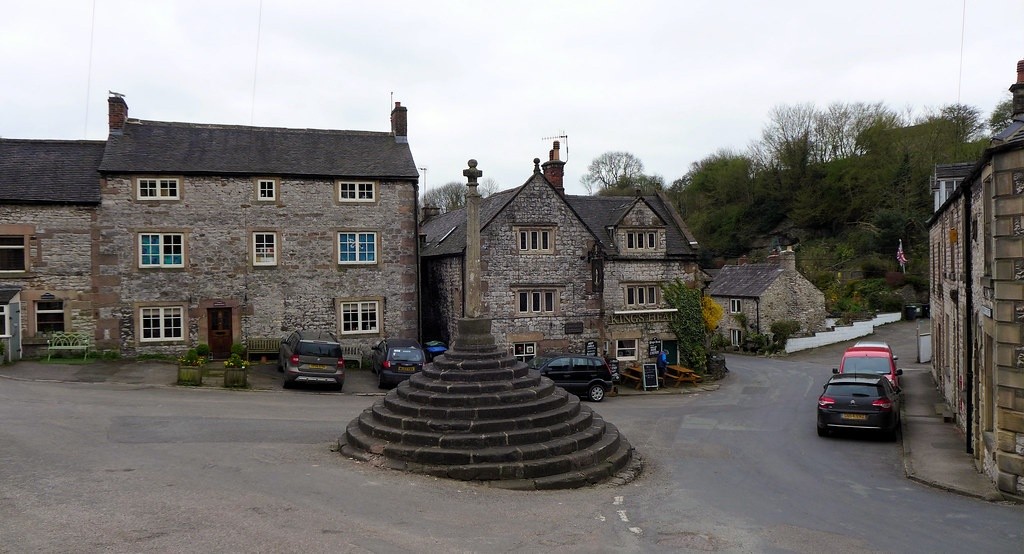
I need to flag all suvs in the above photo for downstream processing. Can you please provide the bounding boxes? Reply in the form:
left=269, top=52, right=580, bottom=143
left=370, top=338, right=426, bottom=388
left=277, top=330, right=345, bottom=391
left=523, top=352, right=614, bottom=402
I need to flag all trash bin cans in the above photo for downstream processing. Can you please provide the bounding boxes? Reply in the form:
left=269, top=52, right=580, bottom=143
left=905, top=302, right=930, bottom=321
left=424, top=340, right=447, bottom=363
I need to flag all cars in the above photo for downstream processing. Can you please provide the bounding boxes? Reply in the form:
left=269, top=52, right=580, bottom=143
left=424, top=343, right=448, bottom=364
left=817, top=372, right=902, bottom=442
left=832, top=346, right=904, bottom=391
left=853, top=341, right=899, bottom=371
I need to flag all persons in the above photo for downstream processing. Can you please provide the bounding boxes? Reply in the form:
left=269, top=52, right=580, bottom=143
left=657, top=349, right=670, bottom=388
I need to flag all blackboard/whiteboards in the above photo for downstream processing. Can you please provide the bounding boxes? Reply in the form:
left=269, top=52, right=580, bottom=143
left=585, top=341, right=598, bottom=358
left=608, top=359, right=621, bottom=381
left=641, top=364, right=658, bottom=387
left=649, top=340, right=661, bottom=356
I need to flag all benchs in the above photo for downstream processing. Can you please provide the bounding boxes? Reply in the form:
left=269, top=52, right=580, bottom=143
left=247, top=338, right=281, bottom=360
left=658, top=376, right=663, bottom=380
left=664, top=372, right=680, bottom=379
left=341, top=345, right=362, bottom=369
left=46, top=332, right=91, bottom=363
left=686, top=372, right=701, bottom=379
left=621, top=372, right=640, bottom=381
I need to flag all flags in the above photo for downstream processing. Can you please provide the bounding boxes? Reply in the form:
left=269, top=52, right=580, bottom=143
left=897, top=241, right=908, bottom=266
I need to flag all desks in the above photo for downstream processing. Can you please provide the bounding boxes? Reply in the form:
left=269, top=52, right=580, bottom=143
left=624, top=367, right=644, bottom=389
left=666, top=365, right=698, bottom=388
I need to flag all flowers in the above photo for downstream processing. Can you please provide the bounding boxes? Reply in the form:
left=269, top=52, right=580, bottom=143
left=178, top=356, right=206, bottom=367
left=223, top=353, right=252, bottom=370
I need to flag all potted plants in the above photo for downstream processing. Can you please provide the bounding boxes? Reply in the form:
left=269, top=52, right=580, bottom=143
left=231, top=343, right=246, bottom=359
left=0, top=341, right=8, bottom=366
left=197, top=344, right=210, bottom=359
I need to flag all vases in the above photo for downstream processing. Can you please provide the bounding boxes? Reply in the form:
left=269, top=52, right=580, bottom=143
left=225, top=367, right=248, bottom=387
left=178, top=366, right=203, bottom=385
